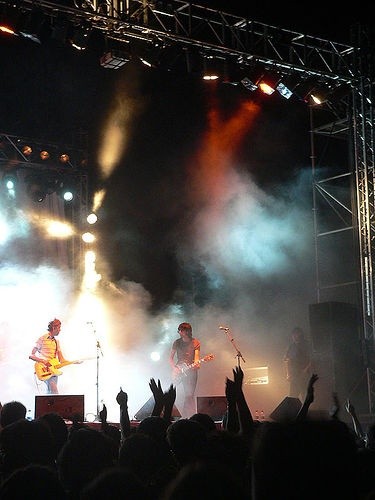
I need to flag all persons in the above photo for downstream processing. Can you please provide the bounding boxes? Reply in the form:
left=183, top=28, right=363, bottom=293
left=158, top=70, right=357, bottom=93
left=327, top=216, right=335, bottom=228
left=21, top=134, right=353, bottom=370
left=0, top=367, right=375, bottom=500
left=169, top=322, right=201, bottom=417
left=29, top=318, right=83, bottom=394
left=284, top=327, right=314, bottom=403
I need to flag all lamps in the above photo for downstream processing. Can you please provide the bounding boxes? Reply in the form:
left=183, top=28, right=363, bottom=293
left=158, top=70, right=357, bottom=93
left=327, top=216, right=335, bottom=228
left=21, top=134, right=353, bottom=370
left=2, top=168, right=98, bottom=225
left=201, top=47, right=337, bottom=107
left=70, top=21, right=160, bottom=72
left=19, top=141, right=71, bottom=165
left=0, top=0, right=50, bottom=43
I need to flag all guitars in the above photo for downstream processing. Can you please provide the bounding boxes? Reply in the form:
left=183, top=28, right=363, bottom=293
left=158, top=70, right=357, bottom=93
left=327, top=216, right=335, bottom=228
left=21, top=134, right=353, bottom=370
left=35, top=355, right=96, bottom=381
left=170, top=353, right=215, bottom=384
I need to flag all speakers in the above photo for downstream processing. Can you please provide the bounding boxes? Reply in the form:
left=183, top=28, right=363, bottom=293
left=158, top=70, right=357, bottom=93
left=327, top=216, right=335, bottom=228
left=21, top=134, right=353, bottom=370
left=35, top=395, right=84, bottom=422
left=134, top=394, right=181, bottom=420
left=309, top=302, right=368, bottom=416
left=197, top=396, right=226, bottom=421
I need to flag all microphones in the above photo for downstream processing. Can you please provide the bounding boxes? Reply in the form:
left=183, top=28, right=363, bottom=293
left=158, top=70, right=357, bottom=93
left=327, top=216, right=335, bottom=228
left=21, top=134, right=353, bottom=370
left=219, top=326, right=229, bottom=330
